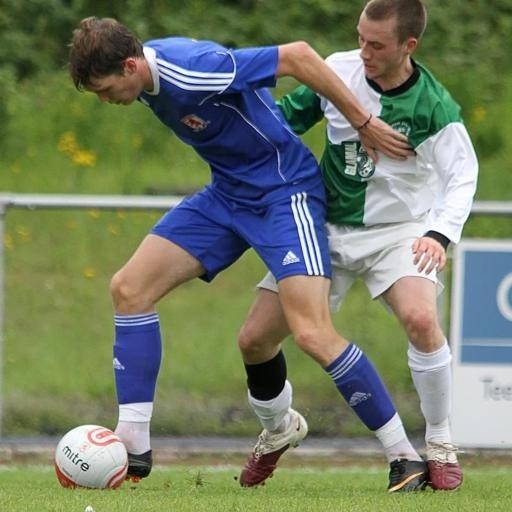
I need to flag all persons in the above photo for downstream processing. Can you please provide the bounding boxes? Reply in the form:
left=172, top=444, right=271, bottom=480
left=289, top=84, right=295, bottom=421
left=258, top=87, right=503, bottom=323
left=68, top=16, right=430, bottom=494
left=239, top=0, right=479, bottom=490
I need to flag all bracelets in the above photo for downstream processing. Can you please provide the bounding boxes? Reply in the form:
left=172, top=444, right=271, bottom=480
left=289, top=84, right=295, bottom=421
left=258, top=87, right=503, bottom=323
left=422, top=230, right=451, bottom=252
left=355, top=113, right=372, bottom=132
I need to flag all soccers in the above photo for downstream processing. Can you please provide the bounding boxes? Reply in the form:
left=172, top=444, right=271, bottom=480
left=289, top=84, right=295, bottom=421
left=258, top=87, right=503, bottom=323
left=54, top=424, right=129, bottom=490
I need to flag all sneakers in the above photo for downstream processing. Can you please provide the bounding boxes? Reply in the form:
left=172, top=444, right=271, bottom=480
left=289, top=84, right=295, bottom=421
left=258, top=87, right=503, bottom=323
left=123, top=449, right=153, bottom=484
left=240, top=409, right=308, bottom=486
left=425, top=438, right=464, bottom=491
left=387, top=456, right=428, bottom=495
